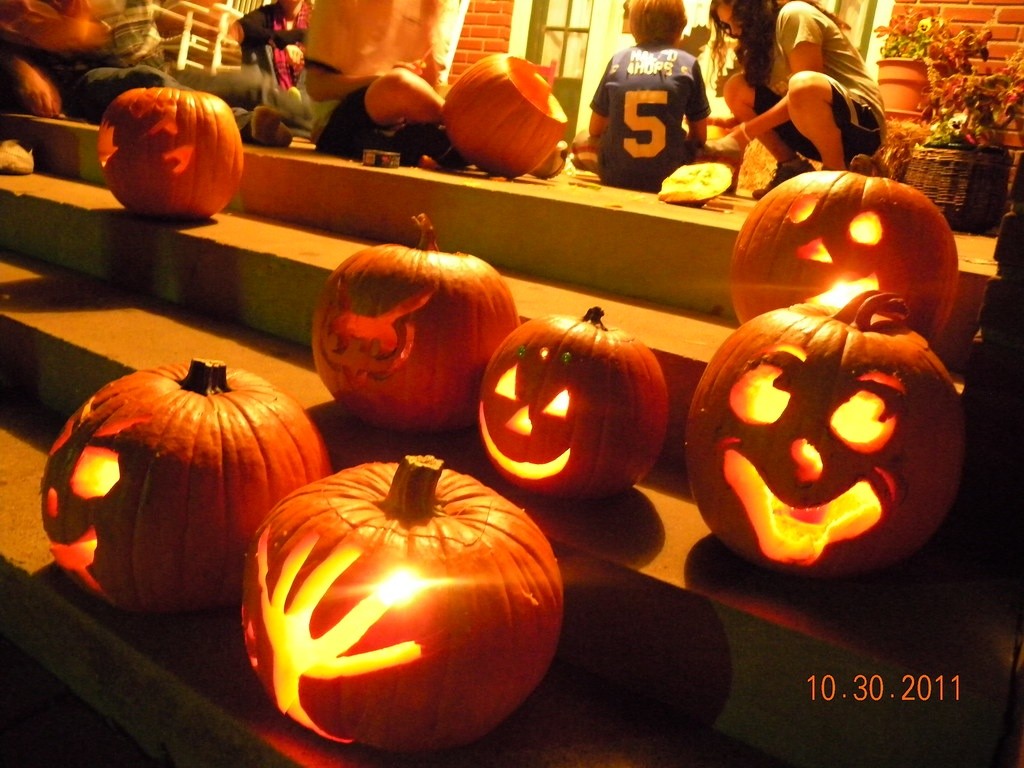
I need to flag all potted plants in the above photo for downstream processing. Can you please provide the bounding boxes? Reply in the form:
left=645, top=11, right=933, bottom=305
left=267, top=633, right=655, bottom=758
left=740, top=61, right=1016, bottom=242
left=909, top=48, right=1023, bottom=232
left=872, top=12, right=994, bottom=121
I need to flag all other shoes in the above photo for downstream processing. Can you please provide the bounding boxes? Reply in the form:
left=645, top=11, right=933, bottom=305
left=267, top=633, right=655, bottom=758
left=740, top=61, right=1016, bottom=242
left=752, top=159, right=816, bottom=201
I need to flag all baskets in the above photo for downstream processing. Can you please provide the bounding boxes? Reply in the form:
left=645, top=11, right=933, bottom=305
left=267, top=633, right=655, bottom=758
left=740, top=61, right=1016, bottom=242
left=902, top=143, right=1013, bottom=235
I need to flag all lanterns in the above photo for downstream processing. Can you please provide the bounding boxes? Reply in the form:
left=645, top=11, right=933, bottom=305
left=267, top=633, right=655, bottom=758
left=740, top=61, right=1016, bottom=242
left=243, top=454, right=566, bottom=752
left=38, top=357, right=324, bottom=615
left=98, top=87, right=245, bottom=223
left=477, top=308, right=665, bottom=505
left=728, top=157, right=958, bottom=345
left=687, top=289, right=959, bottom=584
left=312, top=212, right=517, bottom=441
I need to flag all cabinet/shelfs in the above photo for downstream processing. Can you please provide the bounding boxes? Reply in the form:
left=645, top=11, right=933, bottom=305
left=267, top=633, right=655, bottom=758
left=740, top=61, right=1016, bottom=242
left=153, top=1, right=253, bottom=75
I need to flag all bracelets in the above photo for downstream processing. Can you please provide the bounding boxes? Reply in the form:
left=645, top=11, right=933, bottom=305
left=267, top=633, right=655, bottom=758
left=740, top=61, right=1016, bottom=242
left=740, top=123, right=752, bottom=143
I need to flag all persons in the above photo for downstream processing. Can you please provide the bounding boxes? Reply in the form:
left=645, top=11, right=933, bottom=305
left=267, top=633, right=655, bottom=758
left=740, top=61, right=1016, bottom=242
left=686, top=0, right=887, bottom=200
left=0, top=0, right=570, bottom=185
left=573, top=0, right=712, bottom=192
